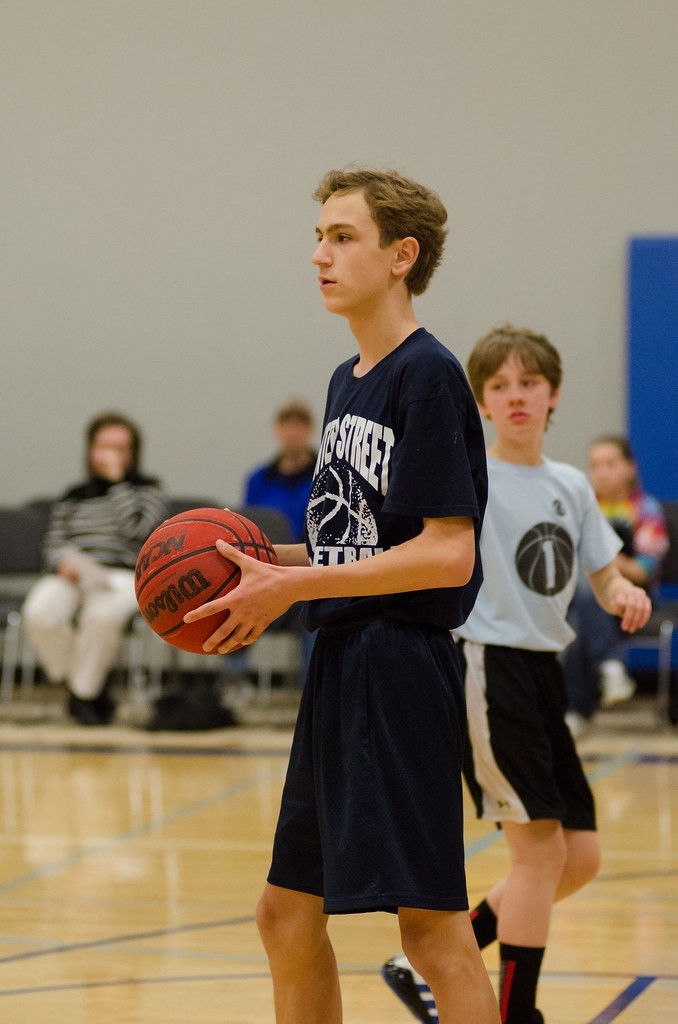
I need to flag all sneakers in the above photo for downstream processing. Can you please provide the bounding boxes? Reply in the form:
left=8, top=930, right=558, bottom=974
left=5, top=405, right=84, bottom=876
left=381, top=950, right=441, bottom=1023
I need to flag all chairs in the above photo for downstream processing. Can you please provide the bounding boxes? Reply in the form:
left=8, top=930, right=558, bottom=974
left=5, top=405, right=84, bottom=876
left=0, top=498, right=296, bottom=726
left=621, top=504, right=678, bottom=730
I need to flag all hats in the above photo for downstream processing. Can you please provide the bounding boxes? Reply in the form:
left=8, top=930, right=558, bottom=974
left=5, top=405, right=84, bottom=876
left=279, top=403, right=308, bottom=423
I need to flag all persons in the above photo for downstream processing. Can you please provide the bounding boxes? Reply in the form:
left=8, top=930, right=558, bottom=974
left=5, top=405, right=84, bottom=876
left=561, top=439, right=667, bottom=736
left=21, top=416, right=170, bottom=727
left=244, top=403, right=317, bottom=675
left=184, top=165, right=502, bottom=1024
left=381, top=328, right=651, bottom=1024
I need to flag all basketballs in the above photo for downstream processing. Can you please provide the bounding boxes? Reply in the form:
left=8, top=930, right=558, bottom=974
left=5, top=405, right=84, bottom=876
left=133, top=507, right=282, bottom=657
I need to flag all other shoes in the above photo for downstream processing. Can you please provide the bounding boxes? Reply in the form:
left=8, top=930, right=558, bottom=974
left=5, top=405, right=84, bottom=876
left=600, top=670, right=636, bottom=706
left=564, top=712, right=590, bottom=740
left=69, top=682, right=117, bottom=727
